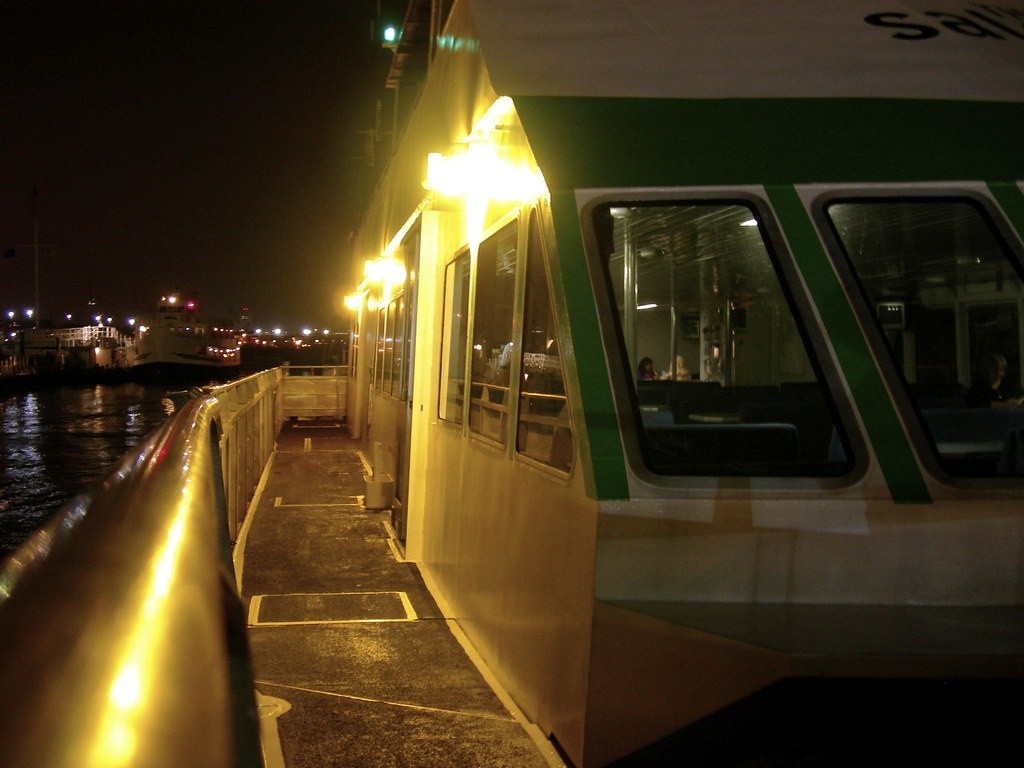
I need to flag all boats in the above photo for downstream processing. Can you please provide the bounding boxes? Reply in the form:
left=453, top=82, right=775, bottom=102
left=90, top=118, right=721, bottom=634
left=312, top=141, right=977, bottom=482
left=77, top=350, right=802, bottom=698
left=126, top=295, right=240, bottom=388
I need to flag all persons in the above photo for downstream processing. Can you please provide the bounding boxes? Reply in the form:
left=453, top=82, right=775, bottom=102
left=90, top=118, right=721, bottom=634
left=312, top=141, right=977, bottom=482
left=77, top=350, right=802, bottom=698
left=978, top=352, right=1009, bottom=402
left=637, top=357, right=660, bottom=380
